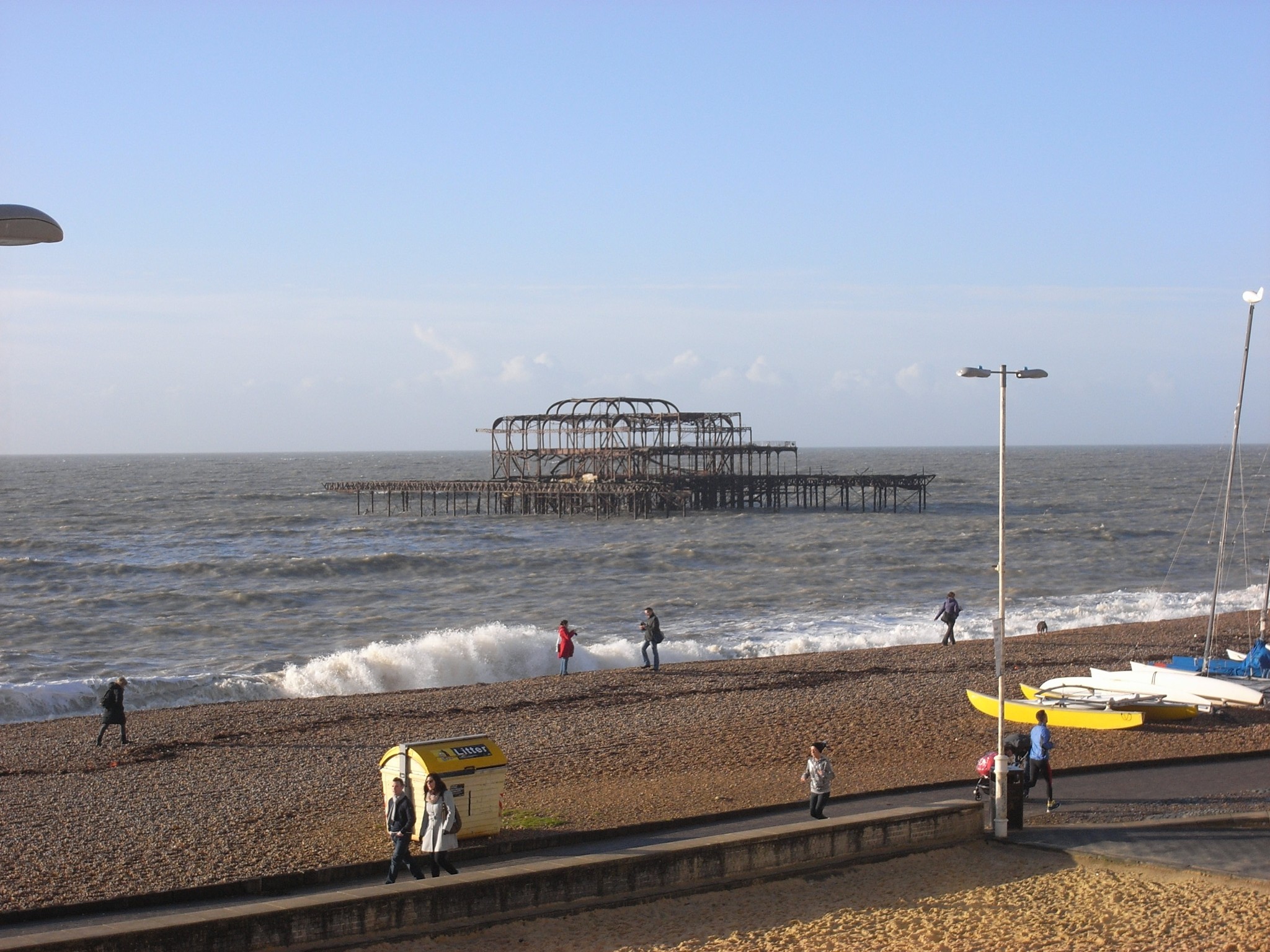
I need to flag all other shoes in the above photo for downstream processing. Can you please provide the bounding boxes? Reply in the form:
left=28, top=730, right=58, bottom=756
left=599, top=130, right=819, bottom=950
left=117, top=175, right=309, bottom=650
left=649, top=668, right=658, bottom=671
left=941, top=642, right=957, bottom=646
left=641, top=665, right=650, bottom=668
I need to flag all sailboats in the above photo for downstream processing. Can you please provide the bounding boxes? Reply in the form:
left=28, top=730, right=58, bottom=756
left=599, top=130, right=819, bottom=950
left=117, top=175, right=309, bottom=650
left=1040, top=287, right=1270, bottom=714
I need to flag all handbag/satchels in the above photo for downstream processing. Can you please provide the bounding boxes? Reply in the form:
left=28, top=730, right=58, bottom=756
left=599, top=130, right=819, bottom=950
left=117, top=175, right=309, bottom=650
left=653, top=632, right=664, bottom=643
left=442, top=790, right=461, bottom=834
left=556, top=644, right=560, bottom=652
left=941, top=612, right=953, bottom=623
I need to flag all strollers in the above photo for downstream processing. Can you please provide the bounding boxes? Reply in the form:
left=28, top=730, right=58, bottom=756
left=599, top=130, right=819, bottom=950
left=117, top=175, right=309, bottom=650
left=972, top=750, right=1031, bottom=801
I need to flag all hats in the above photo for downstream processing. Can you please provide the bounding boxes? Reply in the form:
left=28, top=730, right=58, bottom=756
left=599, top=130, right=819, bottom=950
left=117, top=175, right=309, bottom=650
left=811, top=742, right=826, bottom=754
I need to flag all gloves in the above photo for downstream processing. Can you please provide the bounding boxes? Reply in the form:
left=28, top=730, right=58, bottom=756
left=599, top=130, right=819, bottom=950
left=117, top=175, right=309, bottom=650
left=443, top=830, right=449, bottom=835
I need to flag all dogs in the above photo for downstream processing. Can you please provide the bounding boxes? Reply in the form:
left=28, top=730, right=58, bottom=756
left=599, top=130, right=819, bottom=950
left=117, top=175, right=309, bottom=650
left=1037, top=622, right=1048, bottom=635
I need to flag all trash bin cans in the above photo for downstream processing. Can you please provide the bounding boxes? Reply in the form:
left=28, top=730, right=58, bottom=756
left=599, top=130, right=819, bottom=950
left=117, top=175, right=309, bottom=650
left=377, top=733, right=508, bottom=841
left=989, top=767, right=1023, bottom=829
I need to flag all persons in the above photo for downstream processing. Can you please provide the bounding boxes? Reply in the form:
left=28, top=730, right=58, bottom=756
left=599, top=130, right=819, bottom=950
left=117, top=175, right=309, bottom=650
left=384, top=777, right=425, bottom=884
left=558, top=619, right=577, bottom=676
left=800, top=742, right=835, bottom=820
left=417, top=772, right=462, bottom=878
left=1004, top=710, right=1061, bottom=814
left=639, top=607, right=663, bottom=671
left=934, top=591, right=963, bottom=646
left=95, top=677, right=132, bottom=747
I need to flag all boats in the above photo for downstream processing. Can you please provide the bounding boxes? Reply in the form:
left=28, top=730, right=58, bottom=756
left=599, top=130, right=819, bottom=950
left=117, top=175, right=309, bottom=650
left=963, top=683, right=1200, bottom=729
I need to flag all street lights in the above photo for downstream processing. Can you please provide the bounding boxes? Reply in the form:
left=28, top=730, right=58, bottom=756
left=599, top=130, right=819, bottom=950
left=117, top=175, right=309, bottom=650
left=955, top=365, right=1049, bottom=838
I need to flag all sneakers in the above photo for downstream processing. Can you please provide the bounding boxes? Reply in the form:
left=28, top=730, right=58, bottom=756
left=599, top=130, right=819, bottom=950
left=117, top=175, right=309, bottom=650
left=1047, top=800, right=1061, bottom=812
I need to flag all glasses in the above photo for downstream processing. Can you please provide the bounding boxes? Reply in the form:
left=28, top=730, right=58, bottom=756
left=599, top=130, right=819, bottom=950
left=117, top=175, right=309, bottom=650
left=426, top=779, right=434, bottom=782
